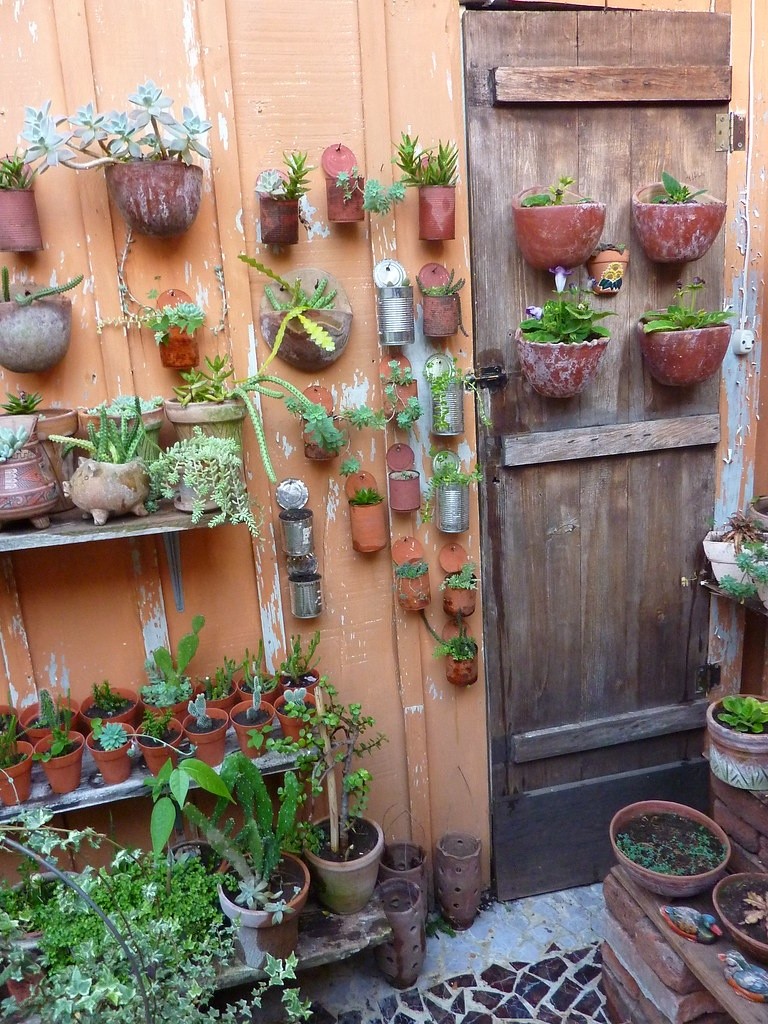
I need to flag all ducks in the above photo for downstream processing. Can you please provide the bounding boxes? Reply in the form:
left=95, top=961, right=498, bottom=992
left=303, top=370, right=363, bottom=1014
left=718, top=950, right=768, bottom=1004
left=662, top=905, right=724, bottom=944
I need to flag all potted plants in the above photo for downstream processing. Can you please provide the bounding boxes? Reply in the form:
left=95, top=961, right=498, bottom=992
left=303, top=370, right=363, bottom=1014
left=0, top=256, right=323, bottom=622
left=389, top=536, right=432, bottom=615
left=431, top=618, right=479, bottom=688
left=514, top=176, right=606, bottom=272
left=385, top=444, right=424, bottom=516
left=415, top=265, right=469, bottom=340
left=703, top=495, right=768, bottom=616
left=421, top=353, right=493, bottom=434
left=345, top=472, right=389, bottom=556
left=1, top=629, right=484, bottom=1024
left=638, top=325, right=731, bottom=386
left=320, top=145, right=404, bottom=224
left=391, top=133, right=461, bottom=242
left=295, top=385, right=340, bottom=462
left=438, top=544, right=481, bottom=620
left=633, top=177, right=727, bottom=264
left=20, top=80, right=214, bottom=237
left=255, top=148, right=317, bottom=246
left=335, top=353, right=424, bottom=433
left=416, top=443, right=483, bottom=535
left=232, top=256, right=354, bottom=389
left=609, top=798, right=768, bottom=956
left=372, top=259, right=416, bottom=348
left=584, top=240, right=630, bottom=296
left=705, top=693, right=767, bottom=790
left=0, top=148, right=43, bottom=252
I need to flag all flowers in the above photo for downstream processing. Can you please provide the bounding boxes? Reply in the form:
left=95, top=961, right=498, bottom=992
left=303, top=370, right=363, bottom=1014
left=517, top=266, right=619, bottom=343
left=639, top=273, right=737, bottom=335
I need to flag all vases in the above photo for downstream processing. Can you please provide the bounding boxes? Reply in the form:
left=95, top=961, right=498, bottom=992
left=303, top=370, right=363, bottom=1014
left=517, top=327, right=609, bottom=400
